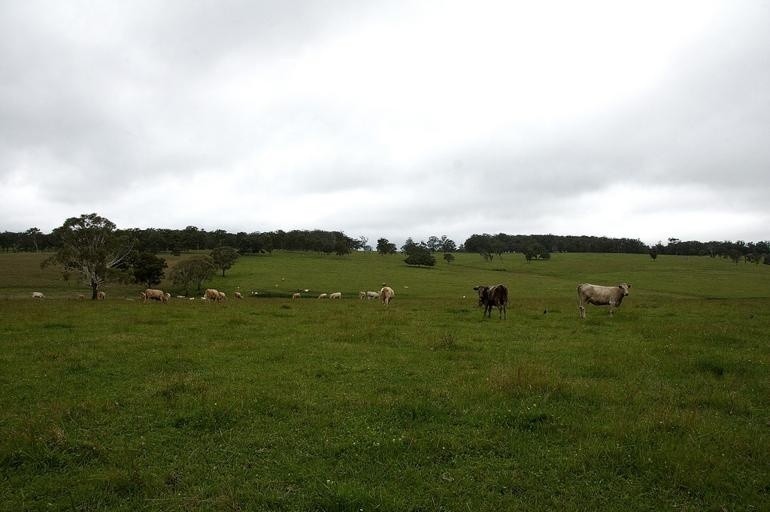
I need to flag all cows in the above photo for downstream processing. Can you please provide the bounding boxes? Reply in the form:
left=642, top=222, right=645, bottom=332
left=32, top=292, right=45, bottom=298
left=140, top=289, right=171, bottom=304
left=329, top=293, right=341, bottom=300
left=359, top=287, right=394, bottom=305
left=576, top=283, right=631, bottom=321
left=473, top=285, right=508, bottom=321
left=203, top=289, right=225, bottom=304
left=292, top=293, right=300, bottom=300
left=98, top=292, right=105, bottom=300
left=318, top=293, right=327, bottom=299
left=235, top=292, right=243, bottom=299
